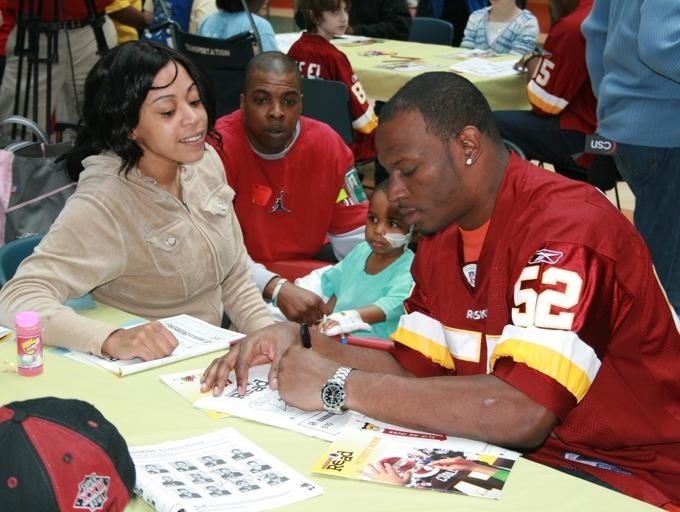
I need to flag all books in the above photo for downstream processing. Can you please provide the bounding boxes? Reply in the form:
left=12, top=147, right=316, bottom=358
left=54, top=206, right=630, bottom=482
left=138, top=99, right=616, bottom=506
left=62, top=313, right=249, bottom=378
left=62, top=313, right=249, bottom=380
left=123, top=427, right=324, bottom=512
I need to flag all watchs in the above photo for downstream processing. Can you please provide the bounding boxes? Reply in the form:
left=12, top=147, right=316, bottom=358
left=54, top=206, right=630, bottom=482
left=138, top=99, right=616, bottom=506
left=321, top=363, right=356, bottom=415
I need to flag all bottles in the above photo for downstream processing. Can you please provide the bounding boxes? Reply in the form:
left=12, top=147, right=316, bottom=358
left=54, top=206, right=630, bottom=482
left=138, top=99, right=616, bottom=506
left=15, top=311, right=45, bottom=377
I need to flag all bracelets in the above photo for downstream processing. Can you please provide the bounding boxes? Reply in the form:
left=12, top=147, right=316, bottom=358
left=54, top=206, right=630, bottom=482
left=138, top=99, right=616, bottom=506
left=272, top=279, right=289, bottom=307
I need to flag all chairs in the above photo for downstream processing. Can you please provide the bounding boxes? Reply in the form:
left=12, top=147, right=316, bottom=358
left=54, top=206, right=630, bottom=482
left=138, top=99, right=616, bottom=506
left=295, top=77, right=354, bottom=145
left=553, top=156, right=622, bottom=215
left=1, top=232, right=98, bottom=311
left=407, top=16, right=455, bottom=44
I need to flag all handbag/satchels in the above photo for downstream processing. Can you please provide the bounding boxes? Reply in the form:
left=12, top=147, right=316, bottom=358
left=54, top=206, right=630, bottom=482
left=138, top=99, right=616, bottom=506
left=1, top=113, right=77, bottom=247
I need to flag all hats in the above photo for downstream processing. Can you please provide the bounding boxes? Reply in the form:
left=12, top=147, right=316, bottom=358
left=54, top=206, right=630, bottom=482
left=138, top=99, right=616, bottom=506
left=0, top=396, right=137, bottom=512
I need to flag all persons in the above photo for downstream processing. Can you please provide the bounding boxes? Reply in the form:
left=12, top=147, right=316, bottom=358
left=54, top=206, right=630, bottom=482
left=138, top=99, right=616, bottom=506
left=0, top=39, right=281, bottom=361
left=144, top=446, right=290, bottom=499
left=105, top=2, right=144, bottom=43
left=580, top=3, right=679, bottom=313
left=1, top=0, right=119, bottom=151
left=460, top=1, right=539, bottom=56
left=196, top=70, right=680, bottom=512
left=345, top=1, right=413, bottom=42
left=140, top=2, right=175, bottom=49
left=292, top=176, right=415, bottom=340
left=415, top=2, right=492, bottom=46
left=288, top=2, right=389, bottom=186
left=188, top=2, right=219, bottom=36
left=491, top=3, right=620, bottom=190
left=0, top=39, right=281, bottom=364
left=358, top=444, right=511, bottom=500
left=196, top=2, right=282, bottom=54
left=205, top=52, right=370, bottom=326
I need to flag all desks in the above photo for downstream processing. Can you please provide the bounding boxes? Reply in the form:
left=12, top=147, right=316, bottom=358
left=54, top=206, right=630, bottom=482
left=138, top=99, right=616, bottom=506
left=275, top=31, right=534, bottom=117
left=0, top=298, right=679, bottom=512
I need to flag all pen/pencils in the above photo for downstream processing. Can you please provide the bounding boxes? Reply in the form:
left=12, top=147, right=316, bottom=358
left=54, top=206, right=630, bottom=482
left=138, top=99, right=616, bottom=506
left=300, top=322, right=311, bottom=349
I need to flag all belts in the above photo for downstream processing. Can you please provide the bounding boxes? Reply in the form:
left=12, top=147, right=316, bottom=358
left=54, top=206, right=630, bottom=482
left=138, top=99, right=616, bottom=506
left=20, top=17, right=94, bottom=31
